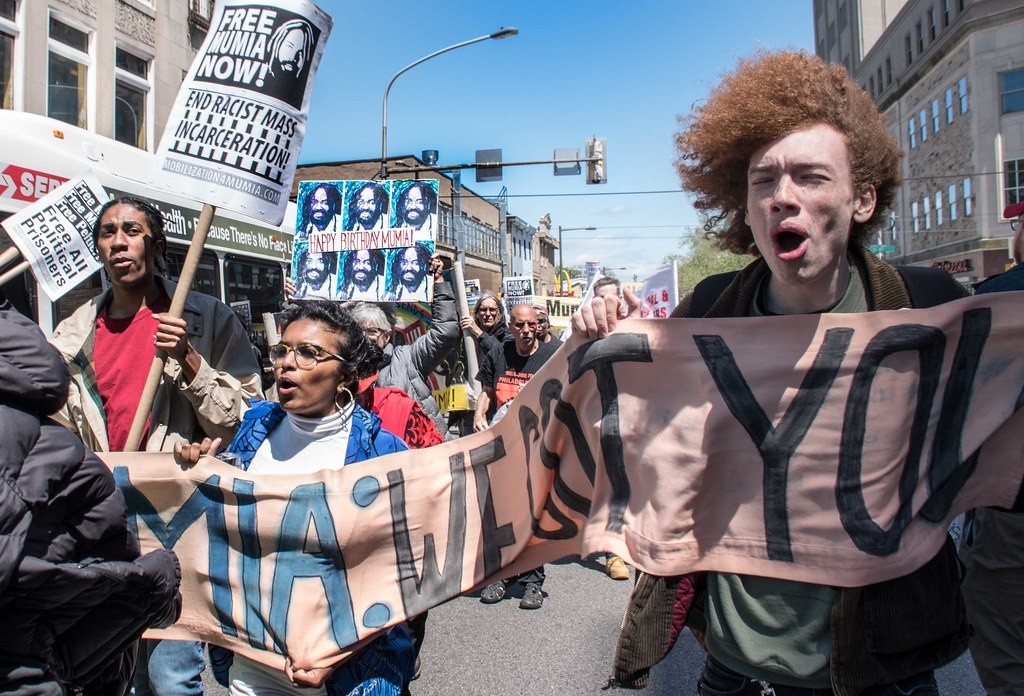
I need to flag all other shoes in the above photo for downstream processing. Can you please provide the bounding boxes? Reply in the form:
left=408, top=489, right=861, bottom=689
left=411, top=655, right=422, bottom=680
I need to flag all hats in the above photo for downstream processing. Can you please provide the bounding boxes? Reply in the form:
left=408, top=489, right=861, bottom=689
left=531, top=304, right=548, bottom=316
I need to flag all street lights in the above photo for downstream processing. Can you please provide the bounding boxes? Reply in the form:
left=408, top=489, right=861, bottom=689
left=603, top=266, right=626, bottom=278
left=378, top=25, right=519, bottom=181
left=558, top=225, right=597, bottom=298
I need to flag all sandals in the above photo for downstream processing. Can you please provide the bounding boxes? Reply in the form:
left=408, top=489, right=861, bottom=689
left=481, top=581, right=506, bottom=603
left=520, top=583, right=543, bottom=608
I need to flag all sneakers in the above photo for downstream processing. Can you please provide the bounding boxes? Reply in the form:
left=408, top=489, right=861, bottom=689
left=605, top=557, right=630, bottom=579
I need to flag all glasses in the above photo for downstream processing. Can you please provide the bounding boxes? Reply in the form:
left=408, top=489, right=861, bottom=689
left=479, top=307, right=499, bottom=312
left=538, top=319, right=548, bottom=323
left=368, top=330, right=384, bottom=345
left=269, top=343, right=345, bottom=369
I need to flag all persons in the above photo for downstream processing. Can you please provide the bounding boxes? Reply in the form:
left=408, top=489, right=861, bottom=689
left=572, top=50, right=1024, bottom=696
left=284, top=276, right=629, bottom=679
left=958, top=199, right=1024, bottom=696
left=52, top=197, right=264, bottom=696
left=1, top=301, right=184, bottom=696
left=174, top=302, right=428, bottom=696
